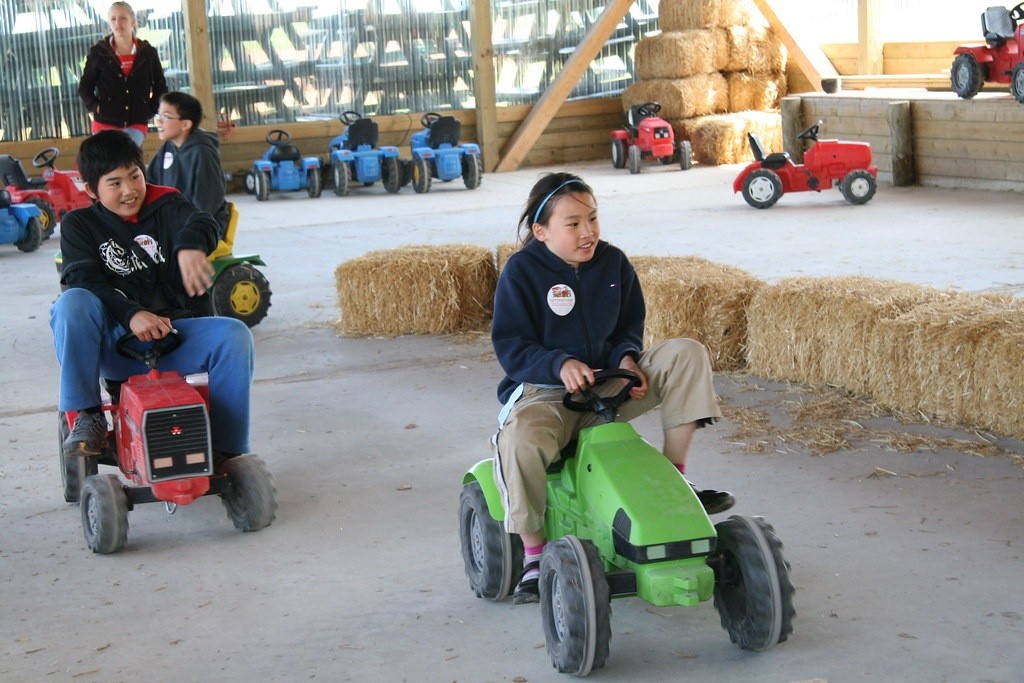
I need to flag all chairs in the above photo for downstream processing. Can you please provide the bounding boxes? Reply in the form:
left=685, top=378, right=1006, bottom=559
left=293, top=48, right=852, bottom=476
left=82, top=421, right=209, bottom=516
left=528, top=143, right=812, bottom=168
left=625, top=105, right=658, bottom=136
left=425, top=116, right=461, bottom=149
left=981, top=6, right=1017, bottom=46
left=343, top=118, right=378, bottom=149
left=268, top=145, right=300, bottom=161
left=747, top=131, right=790, bottom=167
left=0, top=154, right=47, bottom=189
left=207, top=201, right=239, bottom=263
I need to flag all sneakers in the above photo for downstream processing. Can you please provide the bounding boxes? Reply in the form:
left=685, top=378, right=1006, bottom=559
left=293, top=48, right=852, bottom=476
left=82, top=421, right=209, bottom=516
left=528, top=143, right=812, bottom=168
left=63, top=411, right=106, bottom=456
left=213, top=451, right=234, bottom=474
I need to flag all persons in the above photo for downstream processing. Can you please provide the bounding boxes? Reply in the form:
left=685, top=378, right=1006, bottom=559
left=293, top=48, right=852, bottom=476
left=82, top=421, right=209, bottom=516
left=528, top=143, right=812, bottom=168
left=78, top=3, right=170, bottom=163
left=492, top=171, right=735, bottom=603
left=51, top=128, right=255, bottom=477
left=146, top=92, right=231, bottom=247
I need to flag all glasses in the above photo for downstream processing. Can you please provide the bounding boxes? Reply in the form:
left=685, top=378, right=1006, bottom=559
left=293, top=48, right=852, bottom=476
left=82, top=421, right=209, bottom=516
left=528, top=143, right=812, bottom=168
left=153, top=114, right=185, bottom=124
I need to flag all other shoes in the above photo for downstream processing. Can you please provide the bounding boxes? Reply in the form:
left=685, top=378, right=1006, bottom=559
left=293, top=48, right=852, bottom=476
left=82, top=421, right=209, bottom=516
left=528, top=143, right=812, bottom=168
left=697, top=490, right=734, bottom=515
left=513, top=560, right=541, bottom=605
